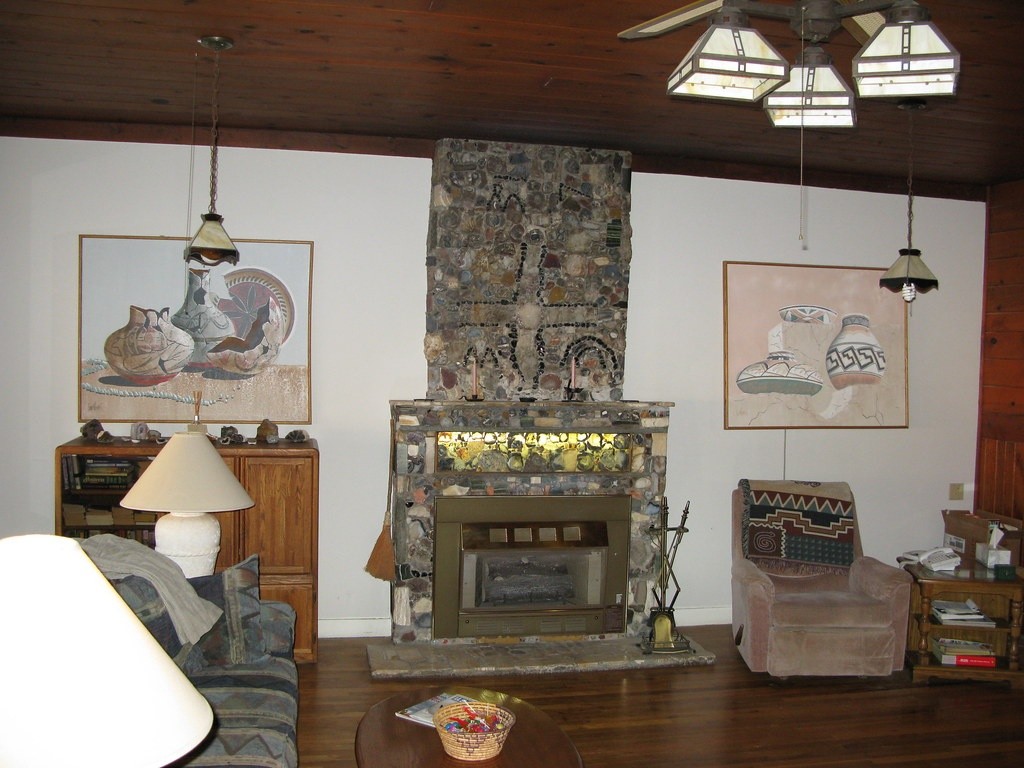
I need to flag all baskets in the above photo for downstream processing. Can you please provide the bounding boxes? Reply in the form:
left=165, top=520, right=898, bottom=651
left=433, top=702, right=517, bottom=762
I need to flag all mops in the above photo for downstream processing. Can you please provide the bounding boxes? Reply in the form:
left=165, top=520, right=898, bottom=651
left=392, top=563, right=411, bottom=627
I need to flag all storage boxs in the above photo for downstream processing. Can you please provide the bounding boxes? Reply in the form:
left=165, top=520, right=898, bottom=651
left=940, top=507, right=1024, bottom=566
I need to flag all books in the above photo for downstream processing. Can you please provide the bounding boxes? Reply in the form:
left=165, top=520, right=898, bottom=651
left=63, top=530, right=156, bottom=550
left=62, top=454, right=136, bottom=490
left=932, top=599, right=996, bottom=627
left=62, top=502, right=169, bottom=525
left=395, top=691, right=478, bottom=727
left=931, top=637, right=996, bottom=667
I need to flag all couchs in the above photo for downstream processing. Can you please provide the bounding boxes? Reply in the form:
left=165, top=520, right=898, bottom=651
left=732, top=479, right=912, bottom=676
left=73, top=534, right=297, bottom=768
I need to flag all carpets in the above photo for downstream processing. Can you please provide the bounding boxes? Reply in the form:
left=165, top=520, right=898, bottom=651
left=367, top=628, right=717, bottom=679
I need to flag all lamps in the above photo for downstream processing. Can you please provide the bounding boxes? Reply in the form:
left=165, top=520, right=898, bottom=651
left=120, top=433, right=254, bottom=577
left=666, top=0, right=959, bottom=127
left=184, top=36, right=239, bottom=265
left=0, top=534, right=214, bottom=768
left=879, top=97, right=939, bottom=303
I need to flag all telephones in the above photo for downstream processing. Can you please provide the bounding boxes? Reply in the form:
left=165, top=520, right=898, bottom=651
left=917, top=547, right=961, bottom=572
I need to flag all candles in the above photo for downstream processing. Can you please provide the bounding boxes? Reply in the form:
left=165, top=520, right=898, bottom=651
left=572, top=356, right=576, bottom=388
left=472, top=361, right=478, bottom=396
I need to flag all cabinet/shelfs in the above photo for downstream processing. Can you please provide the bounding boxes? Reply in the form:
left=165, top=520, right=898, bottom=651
left=56, top=435, right=319, bottom=663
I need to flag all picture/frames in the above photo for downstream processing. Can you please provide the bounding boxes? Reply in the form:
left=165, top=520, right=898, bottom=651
left=723, top=260, right=910, bottom=429
left=79, top=235, right=314, bottom=426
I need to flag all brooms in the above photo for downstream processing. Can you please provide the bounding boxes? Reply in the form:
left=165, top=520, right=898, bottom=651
left=364, top=419, right=396, bottom=582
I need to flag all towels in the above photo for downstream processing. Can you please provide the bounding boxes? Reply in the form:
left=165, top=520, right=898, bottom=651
left=73, top=533, right=224, bottom=646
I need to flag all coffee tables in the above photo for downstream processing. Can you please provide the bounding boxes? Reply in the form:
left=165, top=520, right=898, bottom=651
left=355, top=686, right=582, bottom=768
left=897, top=556, right=1024, bottom=690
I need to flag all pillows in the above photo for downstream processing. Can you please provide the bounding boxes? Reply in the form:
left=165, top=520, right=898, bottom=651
left=186, top=552, right=276, bottom=664
left=109, top=576, right=211, bottom=677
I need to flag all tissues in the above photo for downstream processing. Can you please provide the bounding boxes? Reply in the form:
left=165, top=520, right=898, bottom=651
left=975, top=526, right=1011, bottom=569
